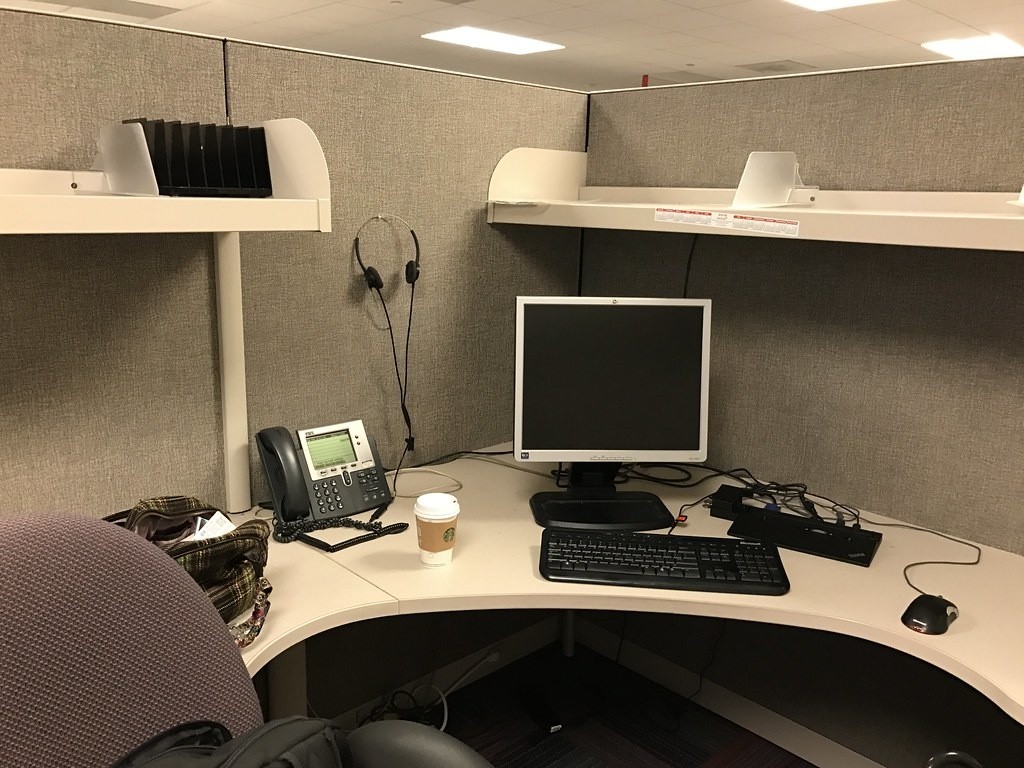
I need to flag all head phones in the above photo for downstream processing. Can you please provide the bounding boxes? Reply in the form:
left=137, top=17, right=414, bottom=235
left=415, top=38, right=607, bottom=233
left=355, top=214, right=420, bottom=290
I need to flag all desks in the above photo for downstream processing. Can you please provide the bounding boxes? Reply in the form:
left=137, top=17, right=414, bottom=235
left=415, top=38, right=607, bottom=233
left=250, top=433, right=1024, bottom=728
left=226, top=513, right=400, bottom=718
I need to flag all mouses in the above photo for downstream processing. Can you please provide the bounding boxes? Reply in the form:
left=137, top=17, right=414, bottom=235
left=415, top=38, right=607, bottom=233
left=901, top=594, right=960, bottom=635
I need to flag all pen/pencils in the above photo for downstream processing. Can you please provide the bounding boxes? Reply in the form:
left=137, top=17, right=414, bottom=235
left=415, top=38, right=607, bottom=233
left=368, top=491, right=397, bottom=524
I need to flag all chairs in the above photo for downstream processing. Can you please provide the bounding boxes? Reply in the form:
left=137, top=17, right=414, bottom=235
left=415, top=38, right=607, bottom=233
left=0, top=511, right=492, bottom=768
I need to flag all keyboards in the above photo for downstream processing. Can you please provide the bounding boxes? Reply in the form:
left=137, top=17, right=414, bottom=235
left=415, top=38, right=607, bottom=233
left=727, top=504, right=883, bottom=567
left=538, top=525, right=790, bottom=596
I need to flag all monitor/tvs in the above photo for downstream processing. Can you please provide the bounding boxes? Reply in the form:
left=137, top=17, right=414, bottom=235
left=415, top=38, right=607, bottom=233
left=513, top=297, right=710, bottom=531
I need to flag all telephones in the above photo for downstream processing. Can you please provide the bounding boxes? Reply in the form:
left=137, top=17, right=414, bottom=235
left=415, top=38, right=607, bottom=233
left=254, top=418, right=393, bottom=531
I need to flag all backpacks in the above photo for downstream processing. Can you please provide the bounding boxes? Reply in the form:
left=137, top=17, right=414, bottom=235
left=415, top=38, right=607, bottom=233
left=110, top=715, right=345, bottom=768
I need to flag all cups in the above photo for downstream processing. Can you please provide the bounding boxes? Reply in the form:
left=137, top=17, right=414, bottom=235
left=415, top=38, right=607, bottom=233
left=413, top=493, right=460, bottom=566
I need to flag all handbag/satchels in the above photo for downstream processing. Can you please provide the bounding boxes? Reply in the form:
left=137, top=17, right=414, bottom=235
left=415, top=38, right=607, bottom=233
left=125, top=495, right=274, bottom=649
